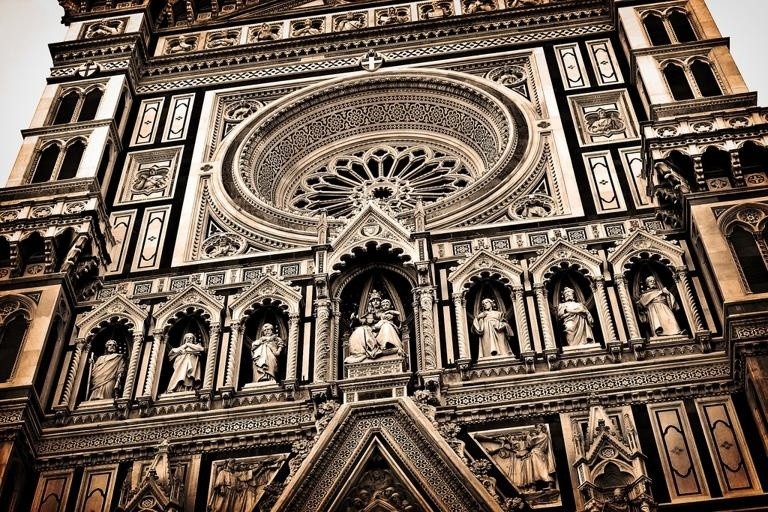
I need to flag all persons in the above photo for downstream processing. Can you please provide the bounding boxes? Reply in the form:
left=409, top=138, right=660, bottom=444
left=252, top=24, right=278, bottom=43
left=336, top=12, right=364, bottom=35
left=135, top=166, right=165, bottom=194
left=422, top=0, right=451, bottom=21
left=170, top=35, right=193, bottom=54
left=637, top=275, right=689, bottom=335
left=591, top=109, right=620, bottom=132
left=294, top=19, right=320, bottom=40
left=554, top=286, right=596, bottom=344
left=204, top=29, right=237, bottom=48
left=206, top=455, right=278, bottom=511
left=83, top=338, right=125, bottom=401
left=250, top=323, right=285, bottom=383
left=382, top=7, right=408, bottom=26
left=371, top=298, right=404, bottom=352
left=167, top=332, right=205, bottom=393
left=469, top=297, right=516, bottom=359
left=342, top=289, right=400, bottom=363
left=494, top=422, right=555, bottom=488
